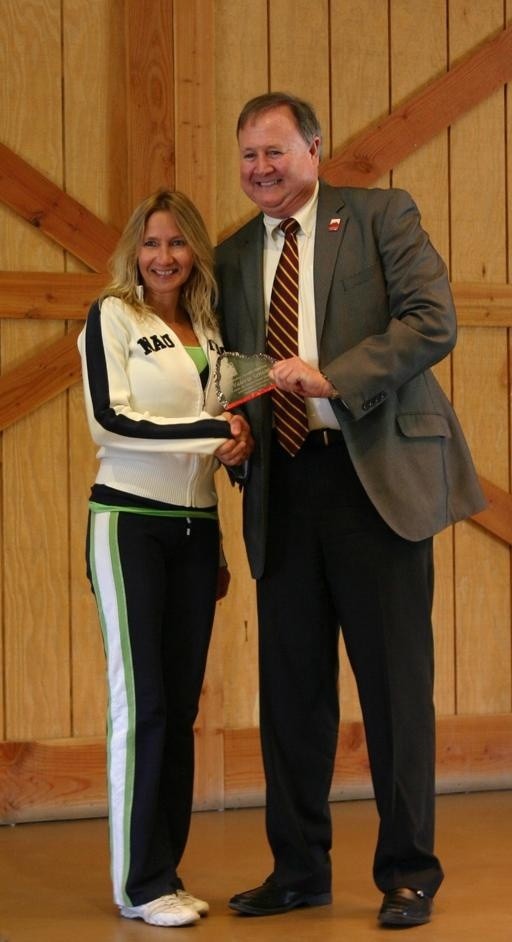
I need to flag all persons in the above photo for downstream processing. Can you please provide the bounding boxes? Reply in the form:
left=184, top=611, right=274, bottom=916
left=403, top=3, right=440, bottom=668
left=76, top=188, right=256, bottom=926
left=212, top=93, right=486, bottom=924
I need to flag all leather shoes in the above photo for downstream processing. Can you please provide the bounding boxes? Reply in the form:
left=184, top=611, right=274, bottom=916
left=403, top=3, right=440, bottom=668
left=229, top=873, right=333, bottom=916
left=378, top=887, right=433, bottom=924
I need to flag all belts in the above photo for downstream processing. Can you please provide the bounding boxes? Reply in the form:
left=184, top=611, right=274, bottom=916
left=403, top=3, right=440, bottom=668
left=271, top=428, right=343, bottom=453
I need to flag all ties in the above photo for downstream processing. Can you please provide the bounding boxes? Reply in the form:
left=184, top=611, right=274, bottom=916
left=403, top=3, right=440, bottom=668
left=266, top=219, right=310, bottom=458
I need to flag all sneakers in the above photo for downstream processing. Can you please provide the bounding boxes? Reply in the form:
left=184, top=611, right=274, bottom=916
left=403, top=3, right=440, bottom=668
left=118, top=887, right=208, bottom=926
